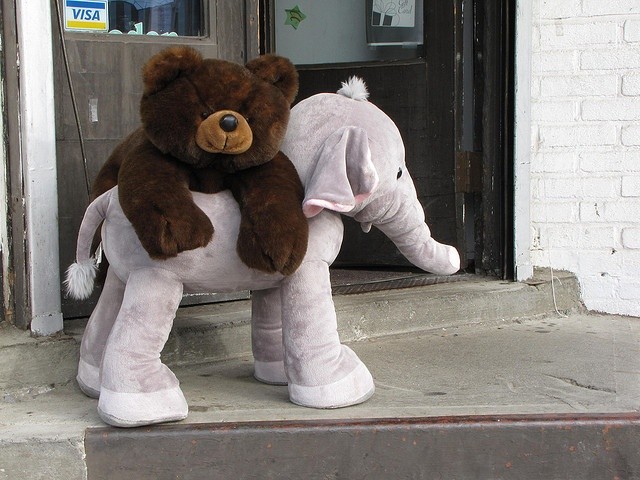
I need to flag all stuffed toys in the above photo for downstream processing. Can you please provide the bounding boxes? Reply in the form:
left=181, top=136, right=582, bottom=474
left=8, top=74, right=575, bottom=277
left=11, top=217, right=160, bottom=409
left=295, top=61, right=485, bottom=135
left=89, top=45, right=309, bottom=277
left=62, top=74, right=462, bottom=428
